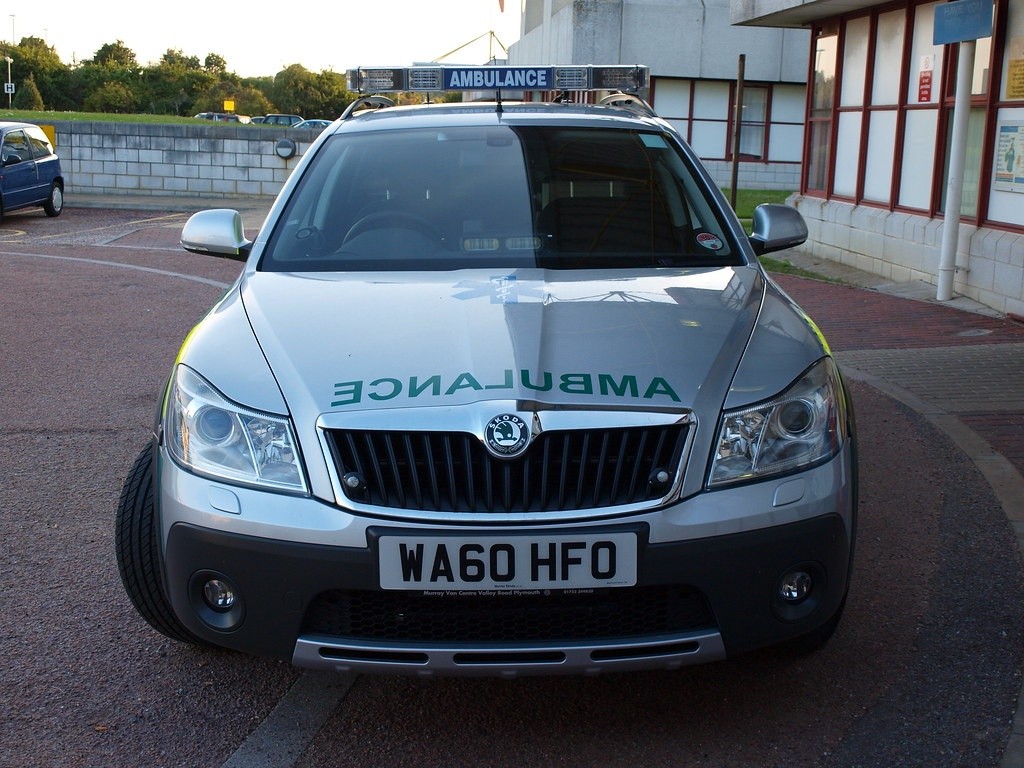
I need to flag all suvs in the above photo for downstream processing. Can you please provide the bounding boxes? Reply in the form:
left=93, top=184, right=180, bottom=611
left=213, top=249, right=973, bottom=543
left=112, top=90, right=857, bottom=681
left=0, top=120, right=65, bottom=219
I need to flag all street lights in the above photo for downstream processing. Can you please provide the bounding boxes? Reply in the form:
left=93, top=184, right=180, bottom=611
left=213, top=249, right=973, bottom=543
left=4, top=56, right=14, bottom=108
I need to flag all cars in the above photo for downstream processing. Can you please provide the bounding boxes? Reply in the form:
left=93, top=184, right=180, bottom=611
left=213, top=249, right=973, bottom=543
left=195, top=110, right=333, bottom=130
